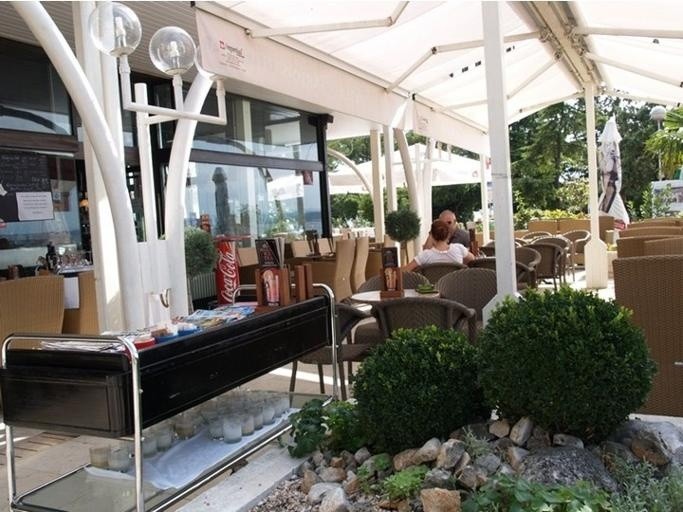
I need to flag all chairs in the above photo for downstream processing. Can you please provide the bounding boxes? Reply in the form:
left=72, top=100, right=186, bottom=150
left=438, top=268, right=498, bottom=334
left=485, top=241, right=521, bottom=249
left=289, top=305, right=381, bottom=403
left=627, top=219, right=683, bottom=230
left=367, top=235, right=394, bottom=279
left=294, top=240, right=355, bottom=311
left=614, top=256, right=683, bottom=418
left=559, top=220, right=590, bottom=234
left=534, top=237, right=572, bottom=285
left=494, top=247, right=540, bottom=289
left=528, top=221, right=557, bottom=235
left=324, top=237, right=369, bottom=294
left=342, top=272, right=431, bottom=382
left=598, top=216, right=614, bottom=241
left=563, top=231, right=591, bottom=281
left=465, top=260, right=537, bottom=292
left=412, top=263, right=468, bottom=289
left=619, top=227, right=683, bottom=237
left=616, top=235, right=683, bottom=255
left=236, top=248, right=264, bottom=300
left=523, top=245, right=564, bottom=292
left=61, top=270, right=99, bottom=336
left=0, top=274, right=64, bottom=365
left=371, top=299, right=477, bottom=351
left=522, top=231, right=550, bottom=239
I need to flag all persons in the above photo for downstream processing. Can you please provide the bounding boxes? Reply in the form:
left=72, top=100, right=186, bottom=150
left=401, top=218, right=476, bottom=271
left=423, top=210, right=470, bottom=252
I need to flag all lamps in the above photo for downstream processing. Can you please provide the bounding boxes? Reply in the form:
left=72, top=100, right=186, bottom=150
left=149, top=26, right=196, bottom=112
left=88, top=2, right=142, bottom=102
left=195, top=45, right=226, bottom=118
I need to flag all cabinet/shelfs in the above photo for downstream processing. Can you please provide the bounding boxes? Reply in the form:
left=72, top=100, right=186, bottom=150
left=1, top=282, right=337, bottom=508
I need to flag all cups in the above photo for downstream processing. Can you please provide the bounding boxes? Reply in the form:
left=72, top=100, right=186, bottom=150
left=471, top=241, right=479, bottom=257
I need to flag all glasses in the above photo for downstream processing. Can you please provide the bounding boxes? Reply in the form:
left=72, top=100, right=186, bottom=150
left=446, top=218, right=457, bottom=224
